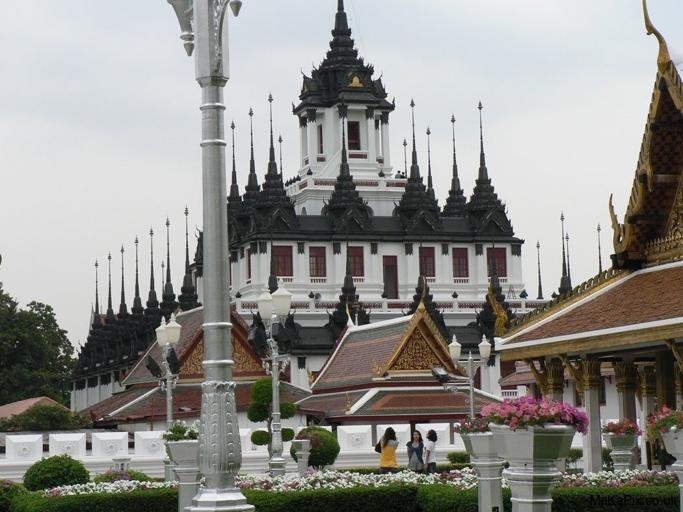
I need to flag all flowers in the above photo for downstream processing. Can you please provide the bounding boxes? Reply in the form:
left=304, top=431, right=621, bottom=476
left=596, top=401, right=682, bottom=445
left=451, top=393, right=589, bottom=435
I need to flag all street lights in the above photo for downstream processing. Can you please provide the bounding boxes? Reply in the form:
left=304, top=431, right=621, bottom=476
left=247, top=278, right=298, bottom=477
left=143, top=312, right=182, bottom=433
left=447, top=333, right=492, bottom=426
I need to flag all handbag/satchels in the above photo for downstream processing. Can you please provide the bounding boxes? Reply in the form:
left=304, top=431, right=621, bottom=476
left=375, top=441, right=381, bottom=453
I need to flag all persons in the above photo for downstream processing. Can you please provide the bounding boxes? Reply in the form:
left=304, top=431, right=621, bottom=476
left=647, top=413, right=667, bottom=471
left=424, top=429, right=439, bottom=473
left=406, top=430, right=426, bottom=473
left=379, top=427, right=400, bottom=474
